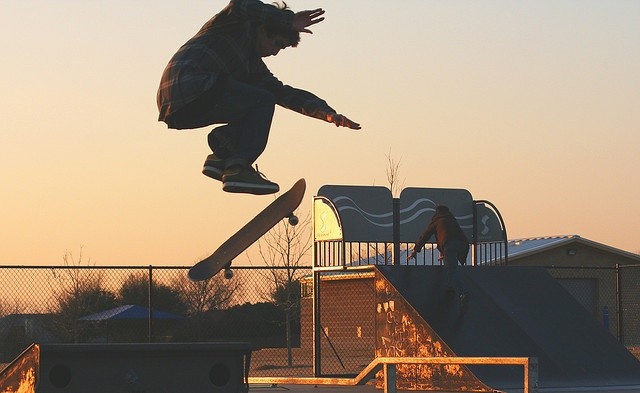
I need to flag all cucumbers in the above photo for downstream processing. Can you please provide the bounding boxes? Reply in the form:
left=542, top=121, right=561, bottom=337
left=188, top=179, right=306, bottom=281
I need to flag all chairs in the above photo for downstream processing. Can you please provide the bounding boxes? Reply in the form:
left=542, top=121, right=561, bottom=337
left=222, top=162, right=279, bottom=195
left=202, top=154, right=225, bottom=180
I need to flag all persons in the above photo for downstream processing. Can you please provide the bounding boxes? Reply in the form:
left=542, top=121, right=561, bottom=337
left=405, top=205, right=471, bottom=306
left=156, top=0, right=361, bottom=195
